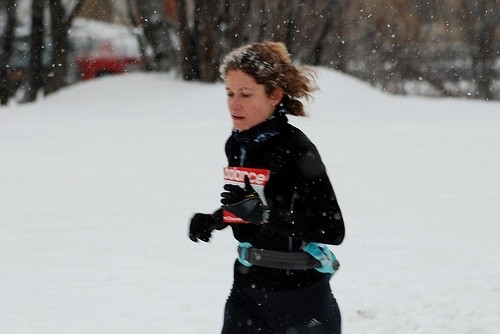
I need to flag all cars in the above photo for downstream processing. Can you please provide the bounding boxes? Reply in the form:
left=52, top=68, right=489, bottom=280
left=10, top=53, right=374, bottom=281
left=75, top=41, right=149, bottom=80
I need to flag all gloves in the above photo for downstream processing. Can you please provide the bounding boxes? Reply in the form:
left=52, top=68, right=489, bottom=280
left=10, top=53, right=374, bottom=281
left=221, top=175, right=272, bottom=225
left=189, top=208, right=229, bottom=242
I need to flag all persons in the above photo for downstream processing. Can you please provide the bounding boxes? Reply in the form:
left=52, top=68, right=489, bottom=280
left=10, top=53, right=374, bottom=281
left=187, top=41, right=345, bottom=333
